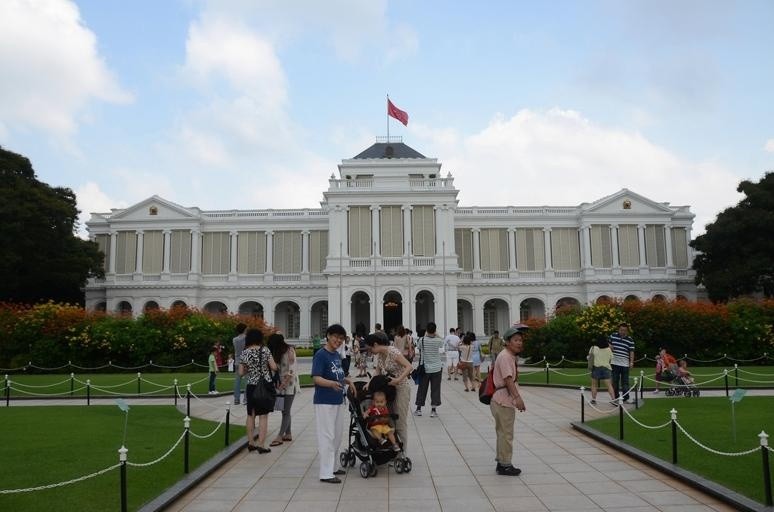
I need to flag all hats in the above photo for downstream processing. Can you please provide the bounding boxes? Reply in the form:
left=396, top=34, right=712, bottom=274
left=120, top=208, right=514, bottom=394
left=503, top=328, right=523, bottom=340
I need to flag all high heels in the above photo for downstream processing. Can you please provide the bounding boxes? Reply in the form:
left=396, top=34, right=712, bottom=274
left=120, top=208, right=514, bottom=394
left=257, top=446, right=271, bottom=454
left=248, top=444, right=257, bottom=452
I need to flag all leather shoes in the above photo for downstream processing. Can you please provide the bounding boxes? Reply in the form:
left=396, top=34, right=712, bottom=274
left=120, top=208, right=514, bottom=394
left=319, top=477, right=342, bottom=483
left=333, top=470, right=346, bottom=475
left=447, top=378, right=452, bottom=380
left=471, top=388, right=475, bottom=391
left=454, top=378, right=458, bottom=380
left=464, top=389, right=469, bottom=391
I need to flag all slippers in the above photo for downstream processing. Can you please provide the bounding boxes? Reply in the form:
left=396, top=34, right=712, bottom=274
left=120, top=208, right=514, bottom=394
left=269, top=441, right=283, bottom=446
left=282, top=436, right=292, bottom=441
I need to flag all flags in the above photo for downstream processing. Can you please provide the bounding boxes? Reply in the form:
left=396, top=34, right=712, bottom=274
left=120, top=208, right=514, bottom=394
left=387, top=96, right=409, bottom=127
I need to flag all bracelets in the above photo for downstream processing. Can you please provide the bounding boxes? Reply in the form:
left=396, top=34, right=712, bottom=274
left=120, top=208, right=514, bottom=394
left=514, top=394, right=520, bottom=400
left=629, top=361, right=633, bottom=363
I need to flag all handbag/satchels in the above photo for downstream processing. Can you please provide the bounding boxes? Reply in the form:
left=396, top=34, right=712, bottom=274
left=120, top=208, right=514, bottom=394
left=587, top=354, right=594, bottom=370
left=254, top=377, right=276, bottom=412
left=273, top=397, right=285, bottom=411
left=661, top=371, right=675, bottom=382
left=479, top=369, right=494, bottom=405
left=403, top=337, right=415, bottom=360
left=410, top=363, right=428, bottom=385
left=457, top=361, right=468, bottom=369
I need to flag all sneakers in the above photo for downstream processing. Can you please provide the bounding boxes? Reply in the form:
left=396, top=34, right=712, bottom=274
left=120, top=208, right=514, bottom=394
left=496, top=462, right=500, bottom=472
left=361, top=374, right=367, bottom=377
left=413, top=410, right=422, bottom=416
left=610, top=399, right=618, bottom=407
left=208, top=390, right=219, bottom=395
left=589, top=399, right=596, bottom=406
left=623, top=399, right=635, bottom=404
left=430, top=411, right=438, bottom=416
left=393, top=443, right=400, bottom=452
left=653, top=391, right=659, bottom=394
left=356, top=374, right=362, bottom=377
left=378, top=438, right=387, bottom=446
left=498, top=465, right=521, bottom=476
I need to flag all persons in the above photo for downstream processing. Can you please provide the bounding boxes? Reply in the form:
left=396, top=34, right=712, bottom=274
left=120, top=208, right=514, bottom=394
left=238, top=327, right=278, bottom=454
left=340, top=323, right=481, bottom=394
left=269, top=334, right=301, bottom=447
left=652, top=348, right=676, bottom=393
left=227, top=353, right=234, bottom=373
left=211, top=339, right=223, bottom=369
left=208, top=346, right=220, bottom=395
left=608, top=322, right=637, bottom=405
left=411, top=321, right=444, bottom=418
left=676, top=359, right=697, bottom=390
left=231, top=322, right=250, bottom=406
left=587, top=335, right=620, bottom=407
left=364, top=391, right=401, bottom=453
left=310, top=324, right=357, bottom=485
left=487, top=330, right=506, bottom=367
left=489, top=326, right=528, bottom=477
left=360, top=333, right=414, bottom=467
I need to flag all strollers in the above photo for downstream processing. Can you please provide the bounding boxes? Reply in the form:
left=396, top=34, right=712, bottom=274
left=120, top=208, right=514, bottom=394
left=662, top=354, right=700, bottom=398
left=338, top=372, right=414, bottom=478
